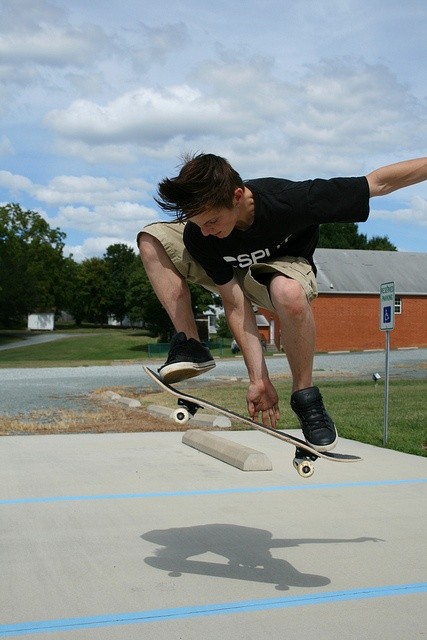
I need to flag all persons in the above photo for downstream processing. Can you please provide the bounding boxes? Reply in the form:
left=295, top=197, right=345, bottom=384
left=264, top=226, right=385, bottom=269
left=136, top=148, right=427, bottom=453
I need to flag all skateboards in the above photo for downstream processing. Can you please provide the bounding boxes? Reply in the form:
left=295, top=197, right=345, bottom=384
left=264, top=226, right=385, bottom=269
left=140, top=365, right=364, bottom=479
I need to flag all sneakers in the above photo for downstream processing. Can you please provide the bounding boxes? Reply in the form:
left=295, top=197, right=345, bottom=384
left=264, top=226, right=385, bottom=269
left=289, top=386, right=339, bottom=452
left=159, top=332, right=216, bottom=383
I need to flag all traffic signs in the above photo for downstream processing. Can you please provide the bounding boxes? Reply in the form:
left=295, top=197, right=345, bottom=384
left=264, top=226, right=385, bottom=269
left=379, top=282, right=396, bottom=332
